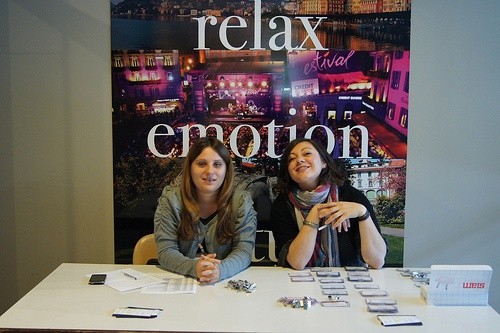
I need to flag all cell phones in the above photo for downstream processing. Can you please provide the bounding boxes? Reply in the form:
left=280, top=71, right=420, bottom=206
left=88, top=273, right=107, bottom=285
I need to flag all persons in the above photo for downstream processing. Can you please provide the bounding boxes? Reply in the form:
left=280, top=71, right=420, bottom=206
left=154, top=136, right=257, bottom=284
left=270, top=138, right=388, bottom=270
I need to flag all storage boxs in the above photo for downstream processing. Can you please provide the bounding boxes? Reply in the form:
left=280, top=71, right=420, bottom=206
left=421, top=264, right=493, bottom=306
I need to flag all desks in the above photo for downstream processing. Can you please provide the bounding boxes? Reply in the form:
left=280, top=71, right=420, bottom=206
left=0, top=262, right=500, bottom=333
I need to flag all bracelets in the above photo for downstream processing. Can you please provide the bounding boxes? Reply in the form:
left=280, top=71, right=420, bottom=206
left=303, top=221, right=319, bottom=228
left=359, top=210, right=369, bottom=221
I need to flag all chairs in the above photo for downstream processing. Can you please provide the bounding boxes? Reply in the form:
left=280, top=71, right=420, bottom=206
left=132, top=234, right=158, bottom=265
